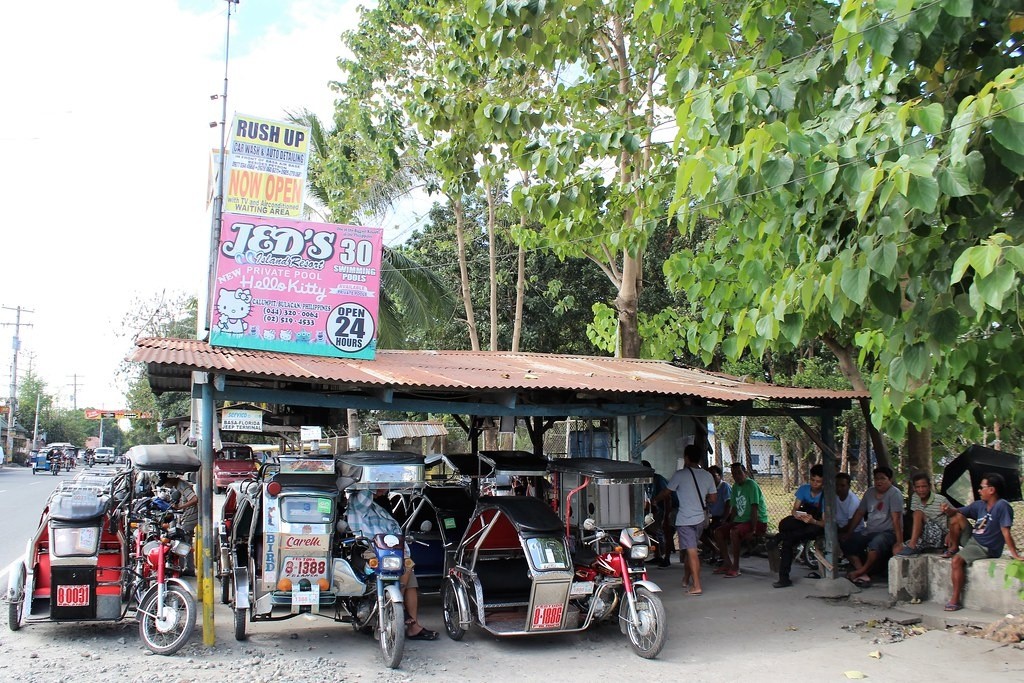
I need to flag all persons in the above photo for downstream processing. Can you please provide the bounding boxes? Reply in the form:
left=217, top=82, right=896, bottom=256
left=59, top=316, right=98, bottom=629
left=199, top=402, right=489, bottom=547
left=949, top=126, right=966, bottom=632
left=701, top=465, right=733, bottom=562
left=841, top=465, right=904, bottom=588
left=652, top=444, right=718, bottom=595
left=772, top=464, right=824, bottom=587
left=155, top=473, right=198, bottom=576
left=896, top=474, right=956, bottom=557
left=941, top=473, right=1024, bottom=611
left=835, top=473, right=865, bottom=533
left=50, top=448, right=74, bottom=470
left=86, top=447, right=95, bottom=461
left=713, top=462, right=768, bottom=577
left=641, top=460, right=680, bottom=569
left=373, top=489, right=439, bottom=640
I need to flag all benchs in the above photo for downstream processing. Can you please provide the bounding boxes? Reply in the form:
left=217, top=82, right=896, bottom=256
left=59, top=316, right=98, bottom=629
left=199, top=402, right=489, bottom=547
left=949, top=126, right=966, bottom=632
left=466, top=551, right=531, bottom=608
left=409, top=539, right=444, bottom=577
left=753, top=529, right=781, bottom=574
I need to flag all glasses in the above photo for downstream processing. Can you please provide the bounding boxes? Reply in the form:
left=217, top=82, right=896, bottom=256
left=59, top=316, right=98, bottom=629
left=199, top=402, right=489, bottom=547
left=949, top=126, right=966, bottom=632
left=979, top=485, right=988, bottom=490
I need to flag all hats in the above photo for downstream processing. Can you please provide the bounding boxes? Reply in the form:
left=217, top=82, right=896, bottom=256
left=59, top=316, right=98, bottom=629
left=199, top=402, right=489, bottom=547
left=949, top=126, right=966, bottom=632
left=155, top=473, right=172, bottom=487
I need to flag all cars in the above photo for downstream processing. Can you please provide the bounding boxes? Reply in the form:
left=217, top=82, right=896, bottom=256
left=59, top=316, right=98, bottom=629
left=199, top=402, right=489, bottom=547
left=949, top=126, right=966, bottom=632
left=93, top=447, right=109, bottom=463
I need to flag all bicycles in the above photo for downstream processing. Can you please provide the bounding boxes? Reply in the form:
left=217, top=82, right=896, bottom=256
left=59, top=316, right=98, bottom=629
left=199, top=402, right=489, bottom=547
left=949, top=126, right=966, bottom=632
left=804, top=538, right=870, bottom=570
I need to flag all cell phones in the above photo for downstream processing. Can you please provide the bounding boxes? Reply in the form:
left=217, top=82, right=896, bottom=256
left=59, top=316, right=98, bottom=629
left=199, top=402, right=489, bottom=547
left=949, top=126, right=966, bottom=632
left=795, top=511, right=807, bottom=515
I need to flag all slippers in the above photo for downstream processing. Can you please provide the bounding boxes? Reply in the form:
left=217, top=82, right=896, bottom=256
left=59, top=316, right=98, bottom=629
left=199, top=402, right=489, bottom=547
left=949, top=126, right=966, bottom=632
left=683, top=589, right=702, bottom=596
left=855, top=579, right=870, bottom=588
left=406, top=628, right=440, bottom=640
left=723, top=570, right=742, bottom=578
left=711, top=566, right=731, bottom=574
left=941, top=549, right=959, bottom=558
left=772, top=580, right=792, bottom=588
left=803, top=572, right=821, bottom=579
left=680, top=577, right=689, bottom=588
left=943, top=602, right=963, bottom=611
left=402, top=609, right=415, bottom=625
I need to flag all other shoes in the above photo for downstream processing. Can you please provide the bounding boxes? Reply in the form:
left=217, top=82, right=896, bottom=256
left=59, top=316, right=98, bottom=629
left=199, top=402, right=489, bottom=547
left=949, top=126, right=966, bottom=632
left=897, top=546, right=920, bottom=556
left=766, top=534, right=783, bottom=550
left=658, top=558, right=670, bottom=568
left=645, top=556, right=663, bottom=565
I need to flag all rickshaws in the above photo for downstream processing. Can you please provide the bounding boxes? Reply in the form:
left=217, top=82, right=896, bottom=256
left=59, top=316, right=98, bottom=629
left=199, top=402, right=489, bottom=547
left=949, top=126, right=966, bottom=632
left=1, top=439, right=668, bottom=670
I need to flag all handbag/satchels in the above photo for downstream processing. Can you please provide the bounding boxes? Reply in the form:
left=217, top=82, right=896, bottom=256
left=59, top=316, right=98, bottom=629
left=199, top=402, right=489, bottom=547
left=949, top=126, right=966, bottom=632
left=703, top=508, right=711, bottom=527
left=797, top=504, right=823, bottom=535
